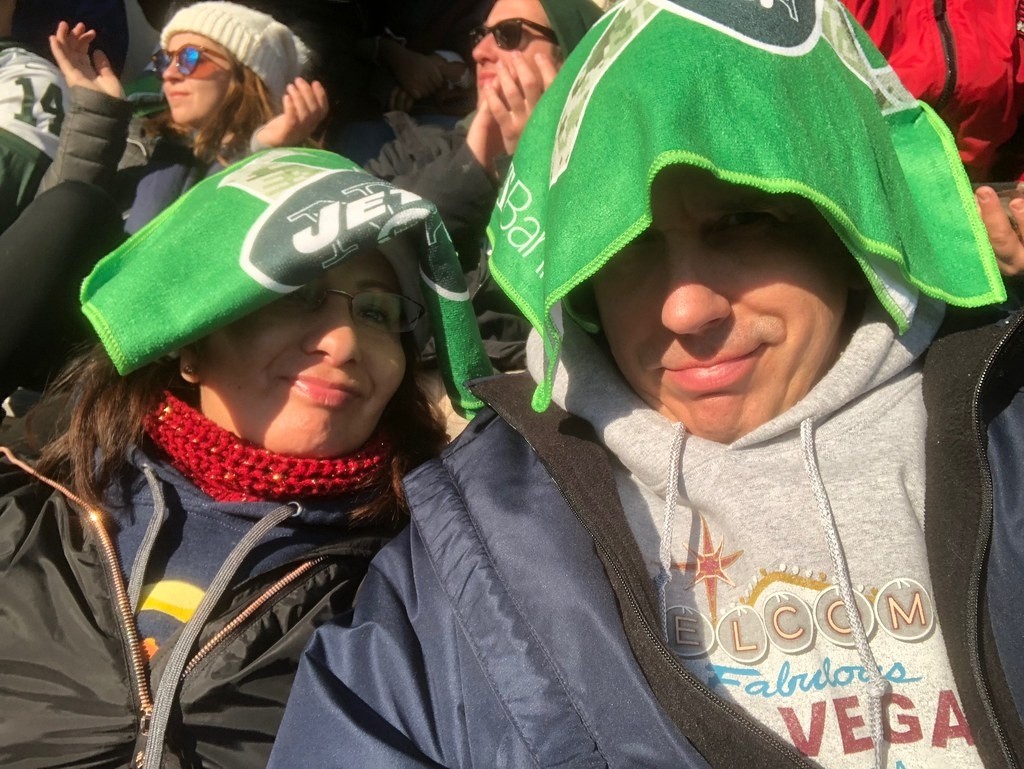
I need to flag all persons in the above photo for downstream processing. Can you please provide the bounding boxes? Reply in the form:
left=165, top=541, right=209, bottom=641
left=0, top=0, right=604, bottom=410
left=0, top=147, right=502, bottom=769
left=264, top=1, right=1024, bottom=769
left=842, top=0, right=1024, bottom=279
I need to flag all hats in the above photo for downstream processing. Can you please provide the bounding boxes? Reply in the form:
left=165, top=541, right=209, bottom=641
left=159, top=1, right=312, bottom=98
left=540, top=0, right=605, bottom=60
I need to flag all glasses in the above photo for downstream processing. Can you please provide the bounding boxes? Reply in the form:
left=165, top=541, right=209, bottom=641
left=266, top=278, right=426, bottom=333
left=151, top=43, right=231, bottom=76
left=467, top=17, right=559, bottom=51
